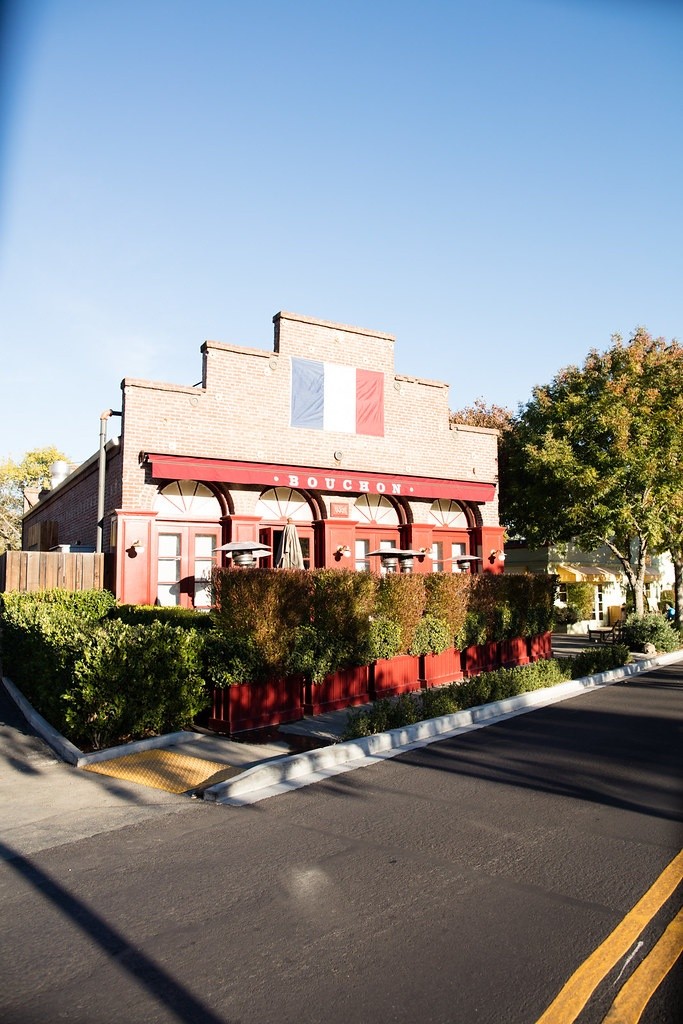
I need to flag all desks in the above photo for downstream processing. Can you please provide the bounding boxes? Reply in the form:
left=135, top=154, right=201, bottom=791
left=588, top=628, right=613, bottom=642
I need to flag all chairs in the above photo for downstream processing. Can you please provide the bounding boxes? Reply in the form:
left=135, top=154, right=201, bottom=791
left=600, top=619, right=626, bottom=645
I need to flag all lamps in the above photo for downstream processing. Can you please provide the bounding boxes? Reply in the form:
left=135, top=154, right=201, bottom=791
left=491, top=548, right=507, bottom=561
left=337, top=544, right=351, bottom=558
left=420, top=547, right=434, bottom=559
left=132, top=539, right=146, bottom=554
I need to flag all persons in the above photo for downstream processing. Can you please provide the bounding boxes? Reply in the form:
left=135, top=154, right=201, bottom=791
left=661, top=604, right=676, bottom=619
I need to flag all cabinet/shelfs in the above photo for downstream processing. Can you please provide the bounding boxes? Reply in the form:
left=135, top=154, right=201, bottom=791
left=608, top=605, right=626, bottom=627
